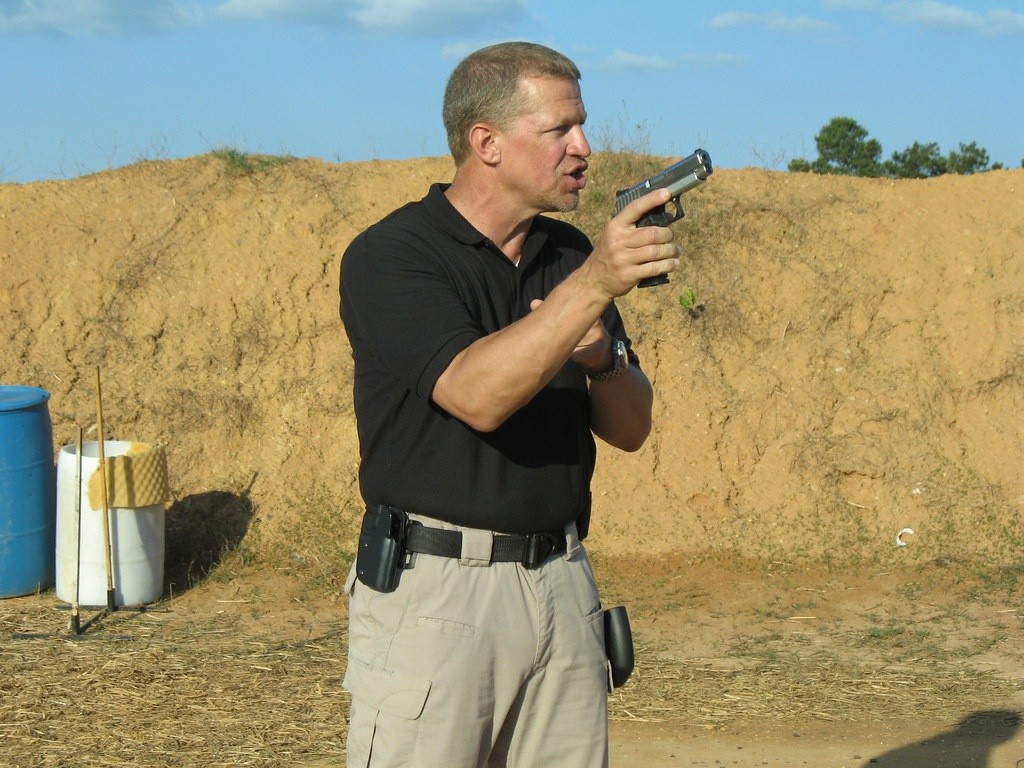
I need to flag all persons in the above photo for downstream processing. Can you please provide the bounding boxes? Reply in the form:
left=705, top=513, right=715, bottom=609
left=338, top=42, right=680, bottom=768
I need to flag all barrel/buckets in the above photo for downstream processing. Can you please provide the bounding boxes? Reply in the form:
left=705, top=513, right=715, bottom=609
left=0, top=386, right=56, bottom=599
left=56, top=440, right=166, bottom=605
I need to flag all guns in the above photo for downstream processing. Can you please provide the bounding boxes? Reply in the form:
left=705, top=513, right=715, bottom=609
left=616, top=148, right=714, bottom=289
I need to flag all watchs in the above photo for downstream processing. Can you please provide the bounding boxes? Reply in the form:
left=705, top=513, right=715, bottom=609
left=586, top=340, right=630, bottom=381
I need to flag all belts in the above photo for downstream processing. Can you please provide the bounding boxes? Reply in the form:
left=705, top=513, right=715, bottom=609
left=403, top=510, right=587, bottom=569
